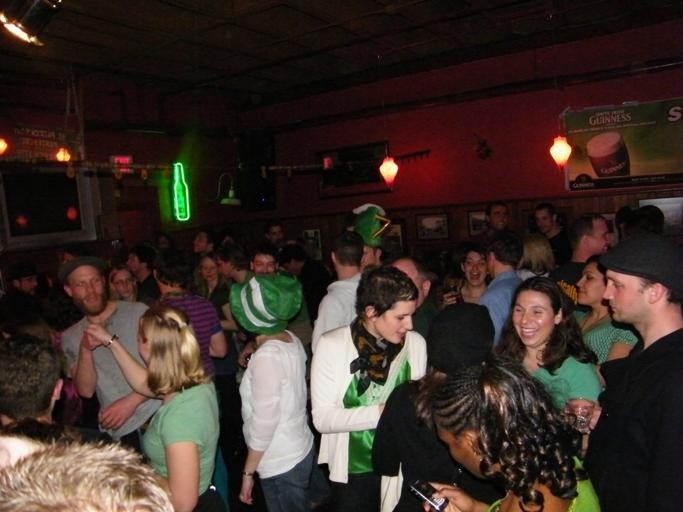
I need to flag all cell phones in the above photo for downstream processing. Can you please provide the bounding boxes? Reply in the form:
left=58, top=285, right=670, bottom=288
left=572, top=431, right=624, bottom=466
left=408, top=477, right=448, bottom=512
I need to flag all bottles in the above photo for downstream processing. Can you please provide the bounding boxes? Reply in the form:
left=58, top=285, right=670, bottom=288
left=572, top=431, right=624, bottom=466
left=170, top=162, right=193, bottom=223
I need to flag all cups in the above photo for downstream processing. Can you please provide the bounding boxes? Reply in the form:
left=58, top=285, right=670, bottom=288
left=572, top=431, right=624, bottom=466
left=444, top=287, right=461, bottom=303
left=565, top=395, right=594, bottom=431
left=586, top=132, right=630, bottom=177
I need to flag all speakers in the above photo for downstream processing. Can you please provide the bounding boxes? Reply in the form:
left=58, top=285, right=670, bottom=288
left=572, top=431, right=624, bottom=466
left=238, top=128, right=276, bottom=212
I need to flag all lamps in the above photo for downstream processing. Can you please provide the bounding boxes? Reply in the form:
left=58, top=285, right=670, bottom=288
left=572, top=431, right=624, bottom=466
left=548, top=105, right=575, bottom=168
left=205, top=171, right=241, bottom=208
left=377, top=142, right=399, bottom=188
left=0, top=1, right=61, bottom=49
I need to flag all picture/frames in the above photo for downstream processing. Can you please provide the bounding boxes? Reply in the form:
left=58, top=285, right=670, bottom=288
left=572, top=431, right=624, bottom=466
left=411, top=211, right=449, bottom=243
left=464, top=209, right=507, bottom=239
left=522, top=207, right=532, bottom=237
left=381, top=217, right=407, bottom=257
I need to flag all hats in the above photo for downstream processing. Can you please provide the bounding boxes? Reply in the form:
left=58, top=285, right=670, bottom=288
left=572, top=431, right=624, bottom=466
left=426, top=301, right=494, bottom=377
left=345, top=202, right=391, bottom=248
left=227, top=271, right=304, bottom=336
left=3, top=260, right=39, bottom=281
left=596, top=231, right=683, bottom=291
